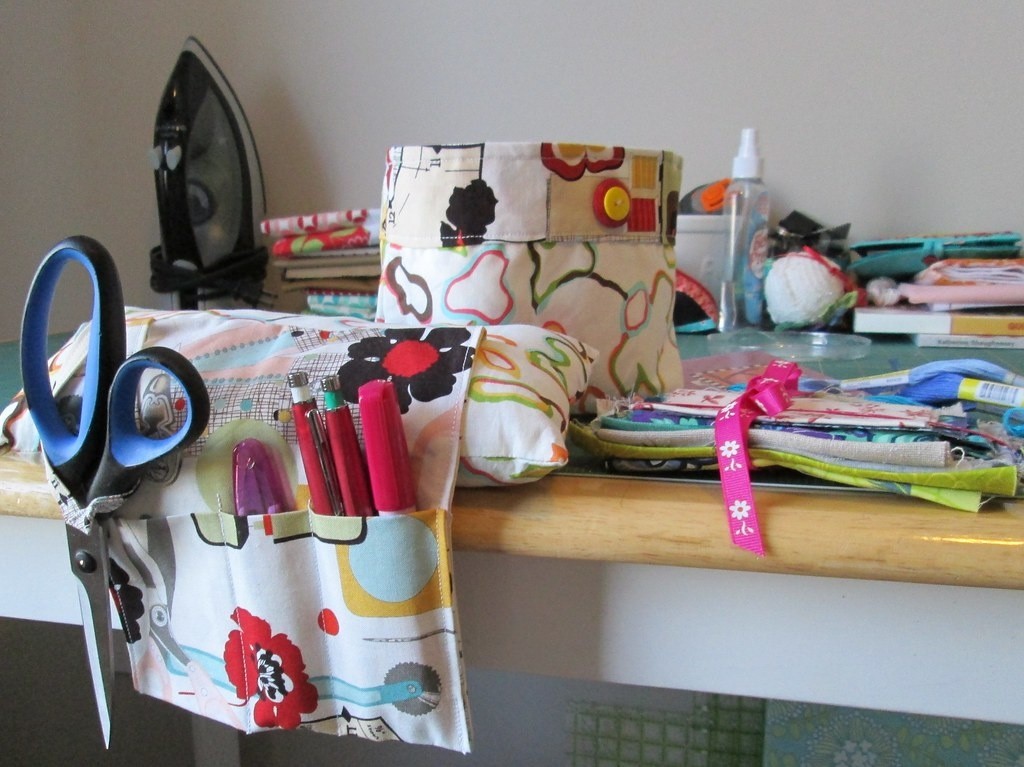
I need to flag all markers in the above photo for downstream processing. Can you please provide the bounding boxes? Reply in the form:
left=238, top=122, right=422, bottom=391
left=357, top=378, right=417, bottom=515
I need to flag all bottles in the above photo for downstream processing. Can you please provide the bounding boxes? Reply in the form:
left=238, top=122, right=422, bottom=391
left=719, top=127, right=772, bottom=334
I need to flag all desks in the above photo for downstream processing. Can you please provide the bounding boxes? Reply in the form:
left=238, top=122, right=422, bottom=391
left=2, top=442, right=1023, bottom=767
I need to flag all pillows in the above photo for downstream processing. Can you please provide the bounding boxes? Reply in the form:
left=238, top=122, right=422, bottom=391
left=1, top=304, right=599, bottom=489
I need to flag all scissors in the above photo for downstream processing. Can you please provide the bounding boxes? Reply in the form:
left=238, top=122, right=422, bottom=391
left=18, top=234, right=213, bottom=752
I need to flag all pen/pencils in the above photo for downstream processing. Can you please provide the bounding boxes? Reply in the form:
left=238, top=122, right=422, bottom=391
left=286, top=369, right=374, bottom=517
